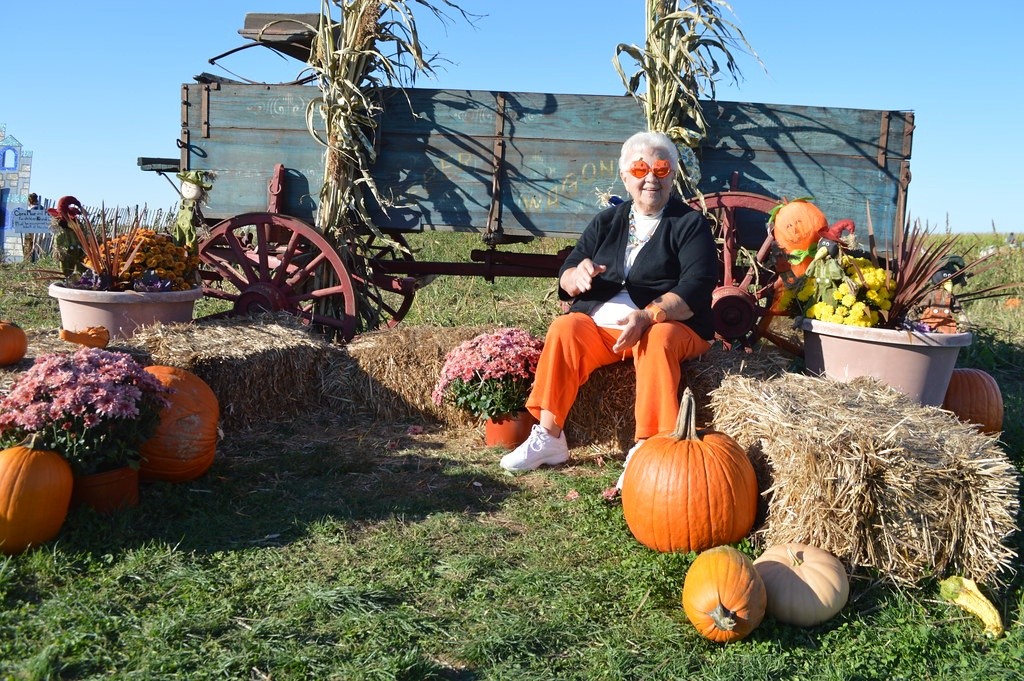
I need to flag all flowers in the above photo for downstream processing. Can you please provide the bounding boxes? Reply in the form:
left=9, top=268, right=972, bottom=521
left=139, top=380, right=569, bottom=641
left=429, top=326, right=544, bottom=417
left=0, top=349, right=171, bottom=466
left=49, top=195, right=201, bottom=290
left=813, top=200, right=1016, bottom=327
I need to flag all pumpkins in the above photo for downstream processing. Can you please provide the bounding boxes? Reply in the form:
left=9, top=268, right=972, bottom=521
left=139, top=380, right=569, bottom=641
left=939, top=575, right=1004, bottom=641
left=941, top=367, right=1004, bottom=439
left=621, top=385, right=757, bottom=552
left=130, top=365, right=224, bottom=483
left=0, top=317, right=28, bottom=365
left=772, top=195, right=828, bottom=282
left=749, top=542, right=848, bottom=628
left=0, top=433, right=72, bottom=553
left=681, top=546, right=767, bottom=643
left=59, top=324, right=110, bottom=349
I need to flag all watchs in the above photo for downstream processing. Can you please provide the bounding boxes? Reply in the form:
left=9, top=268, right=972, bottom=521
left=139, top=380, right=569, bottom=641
left=646, top=304, right=667, bottom=323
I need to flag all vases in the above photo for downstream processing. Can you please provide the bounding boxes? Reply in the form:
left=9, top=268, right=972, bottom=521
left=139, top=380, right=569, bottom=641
left=799, top=317, right=972, bottom=409
left=47, top=282, right=203, bottom=341
left=77, top=468, right=140, bottom=508
left=484, top=409, right=535, bottom=448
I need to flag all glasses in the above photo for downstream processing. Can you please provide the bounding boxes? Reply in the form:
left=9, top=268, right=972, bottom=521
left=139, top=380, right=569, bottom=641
left=625, top=159, right=675, bottom=180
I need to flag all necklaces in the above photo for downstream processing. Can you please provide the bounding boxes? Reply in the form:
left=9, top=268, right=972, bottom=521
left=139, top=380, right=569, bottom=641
left=629, top=208, right=649, bottom=247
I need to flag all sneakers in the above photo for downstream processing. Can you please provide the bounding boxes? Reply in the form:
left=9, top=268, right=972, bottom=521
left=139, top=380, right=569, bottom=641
left=617, top=443, right=643, bottom=489
left=500, top=424, right=569, bottom=472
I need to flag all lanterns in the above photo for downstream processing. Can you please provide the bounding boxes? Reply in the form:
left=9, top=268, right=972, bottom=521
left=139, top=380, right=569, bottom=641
left=773, top=201, right=829, bottom=252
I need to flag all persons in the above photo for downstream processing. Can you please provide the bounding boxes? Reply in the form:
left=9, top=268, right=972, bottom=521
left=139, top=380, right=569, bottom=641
left=499, top=130, right=718, bottom=489
left=47, top=196, right=89, bottom=278
left=172, top=171, right=213, bottom=256
left=28, top=193, right=44, bottom=209
